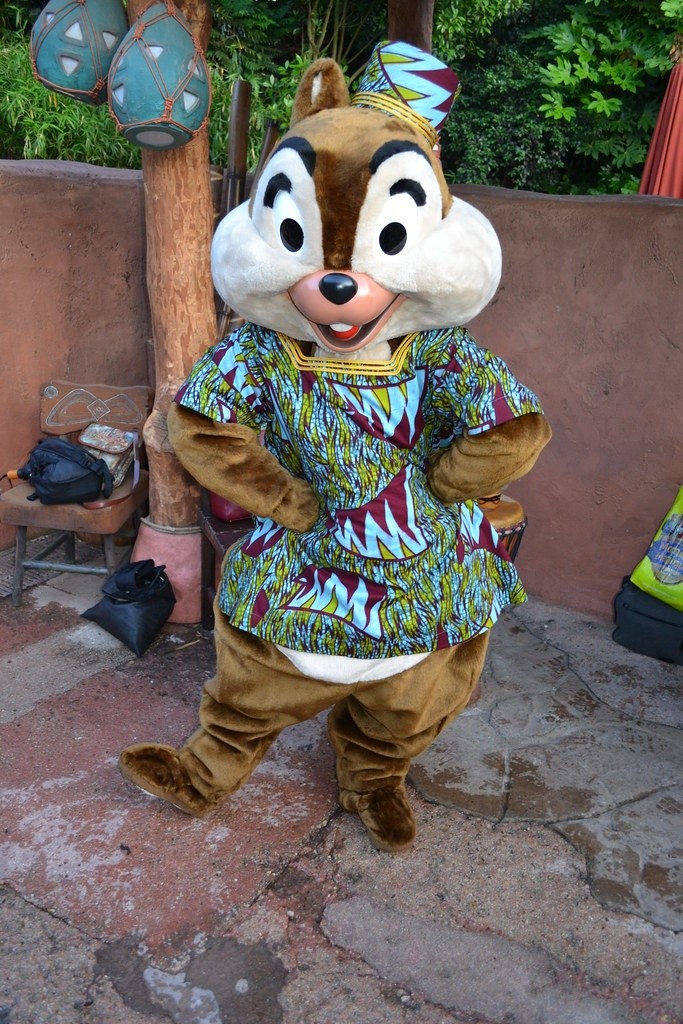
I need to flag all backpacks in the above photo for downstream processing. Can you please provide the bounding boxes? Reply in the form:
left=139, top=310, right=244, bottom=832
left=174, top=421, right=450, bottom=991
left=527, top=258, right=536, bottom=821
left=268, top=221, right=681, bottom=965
left=24, top=438, right=114, bottom=506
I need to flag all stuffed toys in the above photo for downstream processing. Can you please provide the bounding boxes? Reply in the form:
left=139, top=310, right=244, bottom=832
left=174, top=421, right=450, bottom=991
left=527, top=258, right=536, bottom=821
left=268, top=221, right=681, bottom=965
left=112, top=32, right=556, bottom=854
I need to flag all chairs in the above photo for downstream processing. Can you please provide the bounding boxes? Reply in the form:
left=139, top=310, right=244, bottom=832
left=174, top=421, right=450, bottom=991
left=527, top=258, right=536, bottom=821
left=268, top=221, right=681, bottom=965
left=0, top=380, right=154, bottom=607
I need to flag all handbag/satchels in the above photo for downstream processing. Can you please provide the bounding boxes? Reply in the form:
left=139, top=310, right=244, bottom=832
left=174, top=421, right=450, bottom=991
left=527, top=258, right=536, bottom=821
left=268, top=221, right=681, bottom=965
left=81, top=560, right=173, bottom=657
left=76, top=423, right=141, bottom=509
left=612, top=575, right=683, bottom=666
left=630, top=486, right=683, bottom=612
